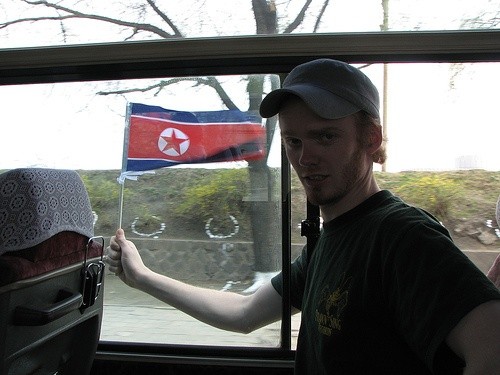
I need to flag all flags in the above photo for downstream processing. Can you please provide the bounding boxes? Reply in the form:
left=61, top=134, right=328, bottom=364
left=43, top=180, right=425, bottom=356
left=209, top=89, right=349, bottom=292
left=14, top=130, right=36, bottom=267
left=116, top=101, right=267, bottom=184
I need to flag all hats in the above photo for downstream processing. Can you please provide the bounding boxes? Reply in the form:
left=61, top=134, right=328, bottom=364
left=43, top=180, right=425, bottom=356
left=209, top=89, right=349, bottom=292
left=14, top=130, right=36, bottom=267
left=259, top=59, right=380, bottom=120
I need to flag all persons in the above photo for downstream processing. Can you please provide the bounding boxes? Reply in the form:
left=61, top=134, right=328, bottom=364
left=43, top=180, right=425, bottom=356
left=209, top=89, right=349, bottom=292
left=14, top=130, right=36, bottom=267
left=105, top=58, right=500, bottom=375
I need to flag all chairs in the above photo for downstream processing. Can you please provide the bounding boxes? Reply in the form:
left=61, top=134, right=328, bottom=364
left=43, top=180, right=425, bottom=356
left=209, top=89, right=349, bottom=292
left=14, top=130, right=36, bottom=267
left=0, top=168, right=107, bottom=375
